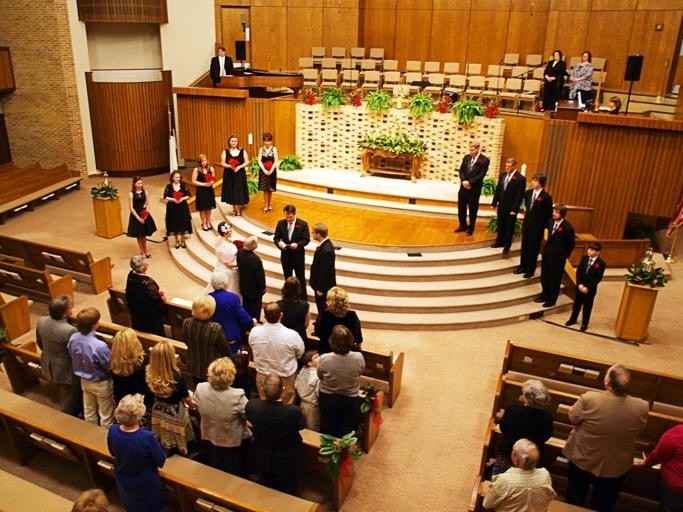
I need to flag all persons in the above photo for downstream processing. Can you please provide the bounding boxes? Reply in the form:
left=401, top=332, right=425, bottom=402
left=560, top=362, right=650, bottom=505
left=563, top=241, right=608, bottom=332
left=485, top=379, right=555, bottom=469
left=454, top=140, right=490, bottom=237
left=481, top=436, right=557, bottom=512
left=202, top=221, right=269, bottom=324
left=306, top=222, right=339, bottom=338
left=67, top=487, right=114, bottom=512
left=160, top=167, right=195, bottom=250
left=123, top=255, right=171, bottom=335
left=32, top=270, right=367, bottom=493
left=535, top=200, right=578, bottom=309
left=607, top=96, right=622, bottom=115
left=566, top=51, right=595, bottom=104
left=104, top=391, right=167, bottom=507
left=271, top=203, right=309, bottom=308
left=540, top=50, right=567, bottom=112
left=633, top=420, right=682, bottom=500
left=216, top=133, right=252, bottom=219
left=192, top=152, right=219, bottom=232
left=125, top=174, right=159, bottom=262
left=489, top=156, right=528, bottom=260
left=209, top=46, right=234, bottom=89
left=513, top=172, right=558, bottom=278
left=255, top=133, right=283, bottom=213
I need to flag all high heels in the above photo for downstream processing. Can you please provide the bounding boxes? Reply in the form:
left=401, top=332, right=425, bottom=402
left=175, top=242, right=179, bottom=249
left=202, top=223, right=211, bottom=231
left=181, top=241, right=186, bottom=248
left=144, top=250, right=151, bottom=258
left=237, top=211, right=241, bottom=218
left=232, top=211, right=237, bottom=216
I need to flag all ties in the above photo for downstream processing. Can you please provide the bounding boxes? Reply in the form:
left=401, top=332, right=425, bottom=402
left=553, top=224, right=557, bottom=230
left=586, top=259, right=592, bottom=272
left=506, top=174, right=510, bottom=182
left=471, top=158, right=474, bottom=165
left=532, top=193, right=536, bottom=202
left=288, top=223, right=293, bottom=241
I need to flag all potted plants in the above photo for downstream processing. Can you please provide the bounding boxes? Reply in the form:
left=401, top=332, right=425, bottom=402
left=358, top=132, right=428, bottom=160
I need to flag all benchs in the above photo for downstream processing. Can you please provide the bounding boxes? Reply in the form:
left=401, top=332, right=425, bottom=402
left=466, top=338, right=682, bottom=511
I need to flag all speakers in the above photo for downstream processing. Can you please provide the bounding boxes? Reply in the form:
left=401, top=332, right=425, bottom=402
left=623, top=54, right=644, bottom=80
left=235, top=40, right=246, bottom=61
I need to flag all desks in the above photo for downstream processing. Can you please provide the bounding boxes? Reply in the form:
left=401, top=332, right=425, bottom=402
left=359, top=147, right=422, bottom=183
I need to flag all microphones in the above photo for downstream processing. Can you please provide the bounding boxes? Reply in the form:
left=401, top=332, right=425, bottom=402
left=546, top=57, right=555, bottom=65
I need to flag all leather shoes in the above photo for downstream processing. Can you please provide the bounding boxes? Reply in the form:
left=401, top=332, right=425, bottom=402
left=535, top=298, right=545, bottom=302
left=513, top=268, right=525, bottom=274
left=581, top=325, right=586, bottom=331
left=523, top=272, right=533, bottom=278
left=269, top=205, right=272, bottom=211
left=491, top=244, right=504, bottom=248
left=263, top=206, right=268, bottom=214
left=466, top=231, right=471, bottom=236
left=566, top=320, right=577, bottom=326
left=455, top=226, right=467, bottom=232
left=543, top=302, right=555, bottom=306
left=503, top=248, right=510, bottom=254
